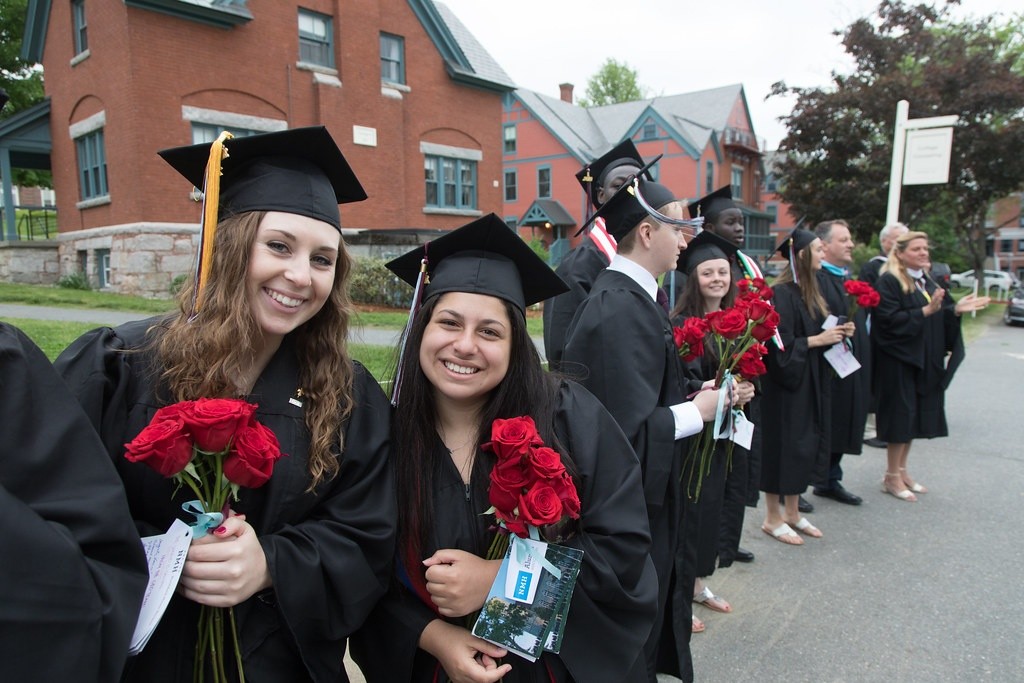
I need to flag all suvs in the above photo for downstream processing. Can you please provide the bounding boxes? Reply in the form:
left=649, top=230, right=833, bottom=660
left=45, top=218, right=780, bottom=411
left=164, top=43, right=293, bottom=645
left=948, top=269, right=1012, bottom=296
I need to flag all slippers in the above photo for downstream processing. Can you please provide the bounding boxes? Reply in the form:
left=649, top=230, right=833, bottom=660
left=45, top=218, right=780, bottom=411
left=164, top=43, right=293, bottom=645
left=783, top=513, right=824, bottom=539
left=761, top=519, right=804, bottom=545
left=692, top=584, right=732, bottom=613
left=690, top=615, right=705, bottom=633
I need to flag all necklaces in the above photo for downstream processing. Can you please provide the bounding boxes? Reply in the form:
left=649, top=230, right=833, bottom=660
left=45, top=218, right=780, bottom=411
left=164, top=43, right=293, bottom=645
left=446, top=441, right=468, bottom=454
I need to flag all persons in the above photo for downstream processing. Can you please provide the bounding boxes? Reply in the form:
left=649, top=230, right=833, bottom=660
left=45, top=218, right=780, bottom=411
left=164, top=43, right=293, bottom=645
left=350, top=215, right=660, bottom=683
left=856, top=223, right=990, bottom=501
left=543, top=139, right=872, bottom=683
left=0, top=323, right=150, bottom=683
left=52, top=127, right=398, bottom=683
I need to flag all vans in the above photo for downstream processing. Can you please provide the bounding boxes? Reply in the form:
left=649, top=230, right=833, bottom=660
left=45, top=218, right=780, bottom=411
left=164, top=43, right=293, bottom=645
left=925, top=262, right=951, bottom=288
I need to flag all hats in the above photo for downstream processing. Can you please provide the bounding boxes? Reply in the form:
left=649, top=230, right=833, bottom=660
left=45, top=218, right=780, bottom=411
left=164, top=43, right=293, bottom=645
left=156, top=124, right=369, bottom=323
left=574, top=136, right=655, bottom=236
left=675, top=230, right=739, bottom=276
left=687, top=182, right=742, bottom=222
left=572, top=152, right=704, bottom=254
left=383, top=211, right=573, bottom=407
left=775, top=213, right=819, bottom=285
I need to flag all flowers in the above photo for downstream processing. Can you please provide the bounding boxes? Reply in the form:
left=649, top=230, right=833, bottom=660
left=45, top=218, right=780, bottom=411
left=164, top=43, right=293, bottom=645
left=121, top=394, right=289, bottom=683
left=944, top=274, right=951, bottom=285
left=830, top=280, right=880, bottom=378
left=671, top=275, right=780, bottom=502
left=469, top=416, right=580, bottom=631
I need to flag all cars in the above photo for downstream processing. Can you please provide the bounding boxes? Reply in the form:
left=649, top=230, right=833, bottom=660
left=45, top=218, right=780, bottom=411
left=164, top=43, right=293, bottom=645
left=1003, top=276, right=1024, bottom=327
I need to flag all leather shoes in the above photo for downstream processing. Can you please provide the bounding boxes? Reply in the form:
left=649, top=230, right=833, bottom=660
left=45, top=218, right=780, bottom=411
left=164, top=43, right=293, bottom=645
left=779, top=489, right=814, bottom=513
left=733, top=547, right=754, bottom=563
left=813, top=477, right=863, bottom=505
left=864, top=438, right=888, bottom=448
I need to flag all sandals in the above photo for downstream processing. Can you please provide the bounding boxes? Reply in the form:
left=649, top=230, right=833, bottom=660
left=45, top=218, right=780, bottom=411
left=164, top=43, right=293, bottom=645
left=900, top=468, right=928, bottom=494
left=881, top=472, right=918, bottom=502
